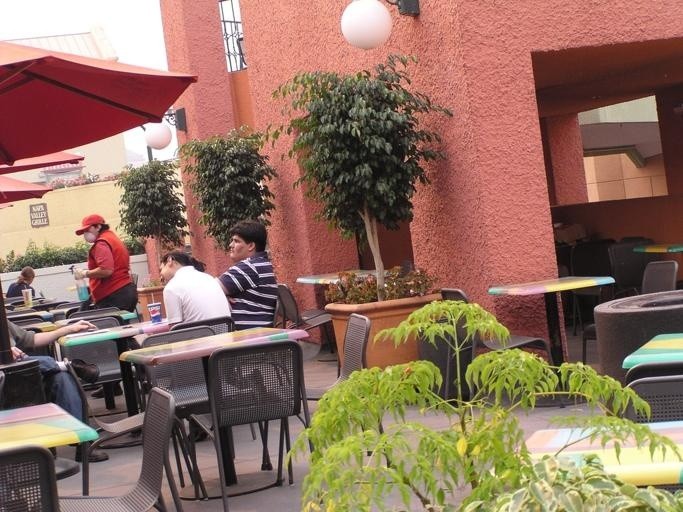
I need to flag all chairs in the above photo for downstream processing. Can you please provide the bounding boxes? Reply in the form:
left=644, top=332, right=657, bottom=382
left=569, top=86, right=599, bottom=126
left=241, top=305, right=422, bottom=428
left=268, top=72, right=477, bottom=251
left=235, top=289, right=292, bottom=389
left=608, top=243, right=649, bottom=300
left=128, top=273, right=143, bottom=322
left=0, top=444, right=59, bottom=511
left=169, top=317, right=257, bottom=440
left=58, top=388, right=183, bottom=512
left=142, top=326, right=273, bottom=484
left=61, top=317, right=145, bottom=420
left=188, top=342, right=316, bottom=512
left=64, top=357, right=185, bottom=496
left=571, top=239, right=615, bottom=336
left=580, top=260, right=679, bottom=405
left=622, top=377, right=683, bottom=424
left=625, top=362, right=683, bottom=389
left=556, top=244, right=573, bottom=327
left=261, top=313, right=392, bottom=489
left=277, top=285, right=335, bottom=373
left=438, top=288, right=564, bottom=420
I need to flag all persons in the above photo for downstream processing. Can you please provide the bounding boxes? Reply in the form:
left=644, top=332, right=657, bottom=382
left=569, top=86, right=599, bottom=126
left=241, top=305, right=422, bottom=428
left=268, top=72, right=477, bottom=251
left=6, top=266, right=35, bottom=296
left=159, top=251, right=231, bottom=442
left=215, top=223, right=278, bottom=327
left=73, top=214, right=152, bottom=397
left=7, top=318, right=110, bottom=462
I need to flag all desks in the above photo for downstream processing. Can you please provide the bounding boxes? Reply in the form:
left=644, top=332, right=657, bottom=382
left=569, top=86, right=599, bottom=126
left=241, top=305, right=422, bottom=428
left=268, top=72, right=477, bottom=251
left=487, top=274, right=611, bottom=408
left=6, top=305, right=92, bottom=323
left=120, top=328, right=308, bottom=500
left=19, top=311, right=138, bottom=418
left=632, top=243, right=682, bottom=253
left=57, top=318, right=169, bottom=449
left=296, top=270, right=387, bottom=288
left=0, top=403, right=99, bottom=497
left=475, top=420, right=683, bottom=496
left=620, top=333, right=683, bottom=370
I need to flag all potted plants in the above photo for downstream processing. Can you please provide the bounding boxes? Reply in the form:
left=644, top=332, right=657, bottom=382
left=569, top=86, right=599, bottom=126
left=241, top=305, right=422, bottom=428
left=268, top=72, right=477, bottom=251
left=272, top=54, right=453, bottom=406
left=113, top=160, right=194, bottom=323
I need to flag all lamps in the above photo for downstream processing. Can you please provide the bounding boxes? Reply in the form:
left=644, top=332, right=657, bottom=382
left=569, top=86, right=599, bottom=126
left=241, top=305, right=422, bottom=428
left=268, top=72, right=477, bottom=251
left=141, top=108, right=187, bottom=150
left=341, top=1, right=419, bottom=50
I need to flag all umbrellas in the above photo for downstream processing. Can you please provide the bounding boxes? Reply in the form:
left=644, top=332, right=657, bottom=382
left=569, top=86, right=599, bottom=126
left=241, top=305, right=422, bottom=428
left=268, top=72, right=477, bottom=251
left=2, top=175, right=53, bottom=203
left=1, top=40, right=197, bottom=365
left=1, top=153, right=83, bottom=172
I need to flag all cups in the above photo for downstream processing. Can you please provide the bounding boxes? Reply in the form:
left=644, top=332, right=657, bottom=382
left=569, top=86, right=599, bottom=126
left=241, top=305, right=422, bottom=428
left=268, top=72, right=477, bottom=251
left=21, top=289, right=32, bottom=307
left=147, top=302, right=162, bottom=324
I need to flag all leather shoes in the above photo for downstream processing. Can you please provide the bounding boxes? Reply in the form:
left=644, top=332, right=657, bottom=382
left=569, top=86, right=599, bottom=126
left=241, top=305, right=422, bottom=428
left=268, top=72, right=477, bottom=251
left=91, top=383, right=123, bottom=397
left=188, top=417, right=213, bottom=444
left=69, top=359, right=100, bottom=384
left=75, top=444, right=109, bottom=463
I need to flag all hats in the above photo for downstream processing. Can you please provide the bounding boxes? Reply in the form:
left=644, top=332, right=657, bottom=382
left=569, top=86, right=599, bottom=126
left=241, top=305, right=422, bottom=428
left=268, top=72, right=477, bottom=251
left=74, top=214, right=106, bottom=235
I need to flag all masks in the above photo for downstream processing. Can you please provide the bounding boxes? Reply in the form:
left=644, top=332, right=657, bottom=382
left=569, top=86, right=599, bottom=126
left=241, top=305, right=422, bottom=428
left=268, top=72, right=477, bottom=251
left=82, top=232, right=96, bottom=243
